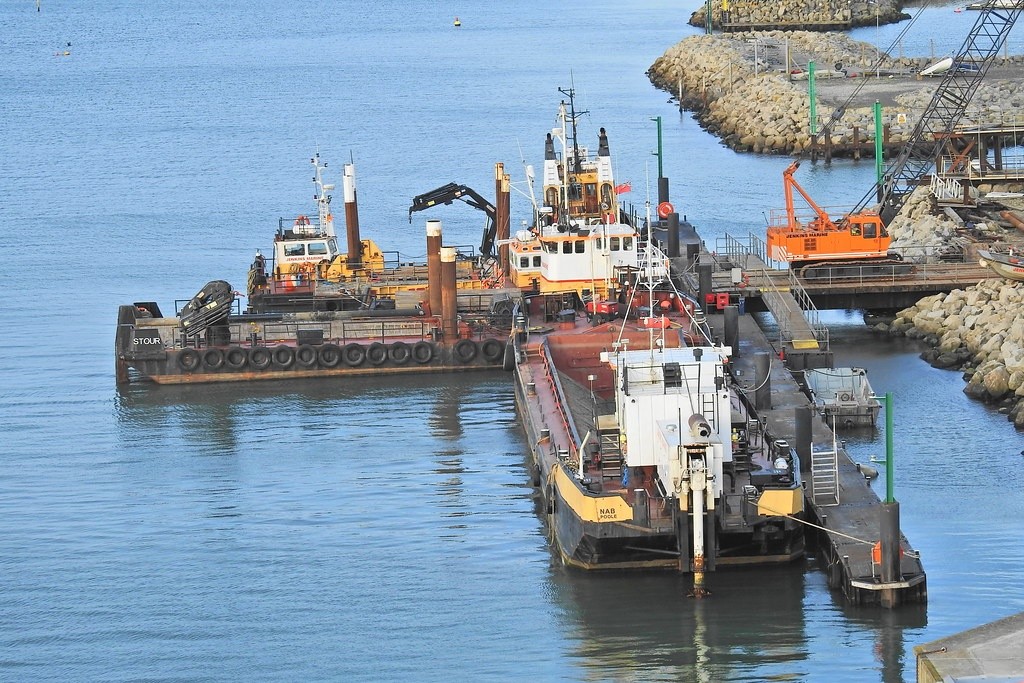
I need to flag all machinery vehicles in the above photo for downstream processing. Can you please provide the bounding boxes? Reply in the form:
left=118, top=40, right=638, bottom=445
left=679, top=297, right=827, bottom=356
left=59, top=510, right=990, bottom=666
left=767, top=0, right=1024, bottom=285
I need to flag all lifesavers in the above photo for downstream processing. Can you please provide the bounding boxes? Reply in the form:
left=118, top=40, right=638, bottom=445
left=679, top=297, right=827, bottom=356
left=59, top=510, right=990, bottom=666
left=452, top=339, right=478, bottom=362
left=300, top=260, right=315, bottom=277
left=295, top=215, right=312, bottom=225
left=479, top=339, right=503, bottom=361
left=501, top=345, right=516, bottom=373
left=175, top=341, right=433, bottom=370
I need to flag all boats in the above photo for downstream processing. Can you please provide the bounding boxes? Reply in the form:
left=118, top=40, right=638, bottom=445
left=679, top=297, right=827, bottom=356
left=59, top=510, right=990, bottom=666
left=114, top=99, right=806, bottom=600
left=954, top=8, right=961, bottom=12
left=977, top=249, right=1024, bottom=282
left=919, top=57, right=954, bottom=75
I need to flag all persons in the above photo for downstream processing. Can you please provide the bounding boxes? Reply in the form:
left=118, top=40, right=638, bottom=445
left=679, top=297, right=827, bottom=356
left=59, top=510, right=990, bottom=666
left=296, top=272, right=303, bottom=284
left=731, top=428, right=741, bottom=453
